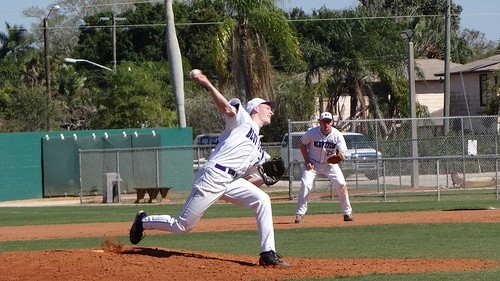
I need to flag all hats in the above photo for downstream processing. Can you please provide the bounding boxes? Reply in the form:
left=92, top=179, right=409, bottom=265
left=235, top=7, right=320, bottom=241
left=245, top=97, right=277, bottom=115
left=320, top=112, right=333, bottom=121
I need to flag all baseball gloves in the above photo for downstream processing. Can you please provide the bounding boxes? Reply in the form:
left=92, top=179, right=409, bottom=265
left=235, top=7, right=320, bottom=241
left=327, top=153, right=343, bottom=164
left=257, top=157, right=285, bottom=186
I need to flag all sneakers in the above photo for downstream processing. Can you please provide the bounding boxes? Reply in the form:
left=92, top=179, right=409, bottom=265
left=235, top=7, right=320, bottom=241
left=129, top=210, right=147, bottom=244
left=259, top=250, right=289, bottom=267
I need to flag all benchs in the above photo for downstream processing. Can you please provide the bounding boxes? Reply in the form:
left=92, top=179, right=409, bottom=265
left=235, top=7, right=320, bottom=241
left=132, top=187, right=172, bottom=203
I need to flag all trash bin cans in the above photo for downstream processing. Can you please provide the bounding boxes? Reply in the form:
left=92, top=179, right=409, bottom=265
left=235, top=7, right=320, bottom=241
left=103, top=173, right=122, bottom=202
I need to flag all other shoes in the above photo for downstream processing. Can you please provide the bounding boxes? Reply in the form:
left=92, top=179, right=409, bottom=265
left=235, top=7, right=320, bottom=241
left=344, top=213, right=353, bottom=221
left=295, top=216, right=304, bottom=224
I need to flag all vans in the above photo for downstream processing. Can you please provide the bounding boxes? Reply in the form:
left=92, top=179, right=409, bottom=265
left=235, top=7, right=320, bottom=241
left=194, top=132, right=222, bottom=150
left=279, top=131, right=383, bottom=181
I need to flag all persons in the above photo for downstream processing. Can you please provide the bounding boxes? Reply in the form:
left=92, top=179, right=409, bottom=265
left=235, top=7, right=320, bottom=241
left=129, top=69, right=292, bottom=267
left=295, top=113, right=354, bottom=222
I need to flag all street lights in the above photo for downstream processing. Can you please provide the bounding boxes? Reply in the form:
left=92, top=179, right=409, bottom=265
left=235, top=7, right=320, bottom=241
left=399, top=28, right=419, bottom=189
left=42, top=5, right=61, bottom=132
left=63, top=14, right=126, bottom=98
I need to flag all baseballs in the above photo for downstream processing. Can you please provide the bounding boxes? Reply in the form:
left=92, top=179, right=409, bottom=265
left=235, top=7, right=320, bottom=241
left=190, top=69, right=202, bottom=80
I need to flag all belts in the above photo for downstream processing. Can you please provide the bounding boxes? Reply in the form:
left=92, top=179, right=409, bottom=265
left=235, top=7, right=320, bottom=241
left=215, top=163, right=237, bottom=178
